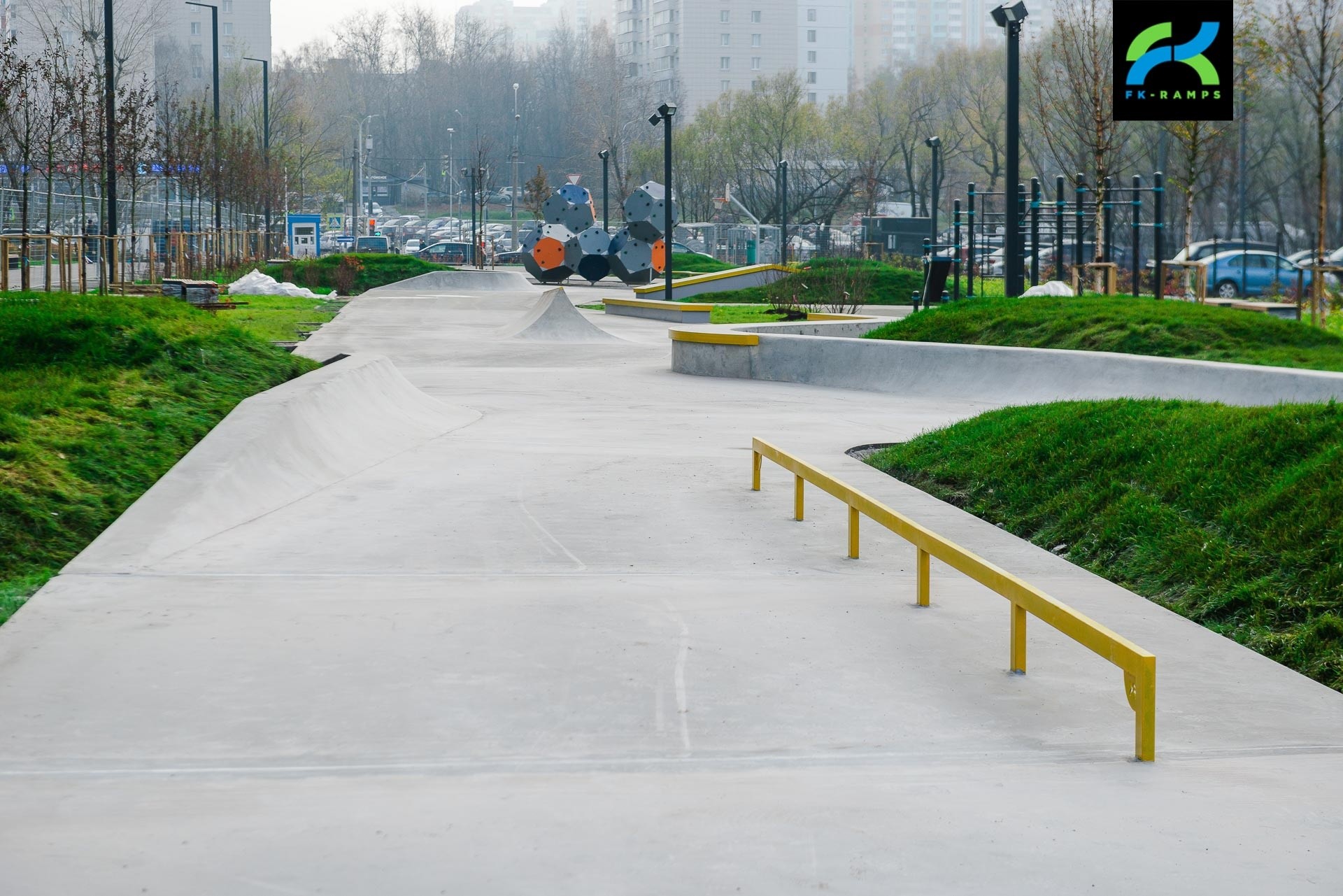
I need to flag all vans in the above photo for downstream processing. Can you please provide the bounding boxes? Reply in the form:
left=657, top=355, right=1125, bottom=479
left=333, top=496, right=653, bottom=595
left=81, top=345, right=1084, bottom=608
left=1, top=227, right=69, bottom=246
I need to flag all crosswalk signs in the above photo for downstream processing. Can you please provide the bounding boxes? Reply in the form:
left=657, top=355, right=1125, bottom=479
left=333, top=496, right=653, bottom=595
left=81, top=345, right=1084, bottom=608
left=329, top=217, right=341, bottom=229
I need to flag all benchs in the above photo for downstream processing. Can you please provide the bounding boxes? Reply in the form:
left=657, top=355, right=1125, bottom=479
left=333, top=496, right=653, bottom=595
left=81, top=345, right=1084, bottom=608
left=1203, top=298, right=1298, bottom=322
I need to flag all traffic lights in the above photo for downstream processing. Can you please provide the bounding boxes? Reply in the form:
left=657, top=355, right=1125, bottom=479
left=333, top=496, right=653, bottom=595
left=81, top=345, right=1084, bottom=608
left=442, top=154, right=449, bottom=171
left=375, top=231, right=381, bottom=235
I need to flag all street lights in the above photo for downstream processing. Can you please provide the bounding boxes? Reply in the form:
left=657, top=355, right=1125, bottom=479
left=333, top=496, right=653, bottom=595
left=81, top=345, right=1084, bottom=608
left=242, top=57, right=271, bottom=262
left=778, top=159, right=788, bottom=266
left=460, top=167, right=486, bottom=267
left=597, top=149, right=610, bottom=234
left=338, top=113, right=379, bottom=214
left=512, top=82, right=521, bottom=252
left=646, top=102, right=677, bottom=299
left=989, top=0, right=1029, bottom=297
left=185, top=1, right=222, bottom=271
left=923, top=136, right=942, bottom=256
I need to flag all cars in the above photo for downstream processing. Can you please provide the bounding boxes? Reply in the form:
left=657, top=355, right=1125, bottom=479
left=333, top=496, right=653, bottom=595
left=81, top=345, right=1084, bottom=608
left=498, top=186, right=529, bottom=199
left=316, top=213, right=545, bottom=264
left=1145, top=238, right=1343, bottom=300
left=786, top=227, right=861, bottom=252
left=935, top=217, right=1149, bottom=277
left=455, top=189, right=510, bottom=204
left=425, top=191, right=457, bottom=204
left=671, top=241, right=712, bottom=258
